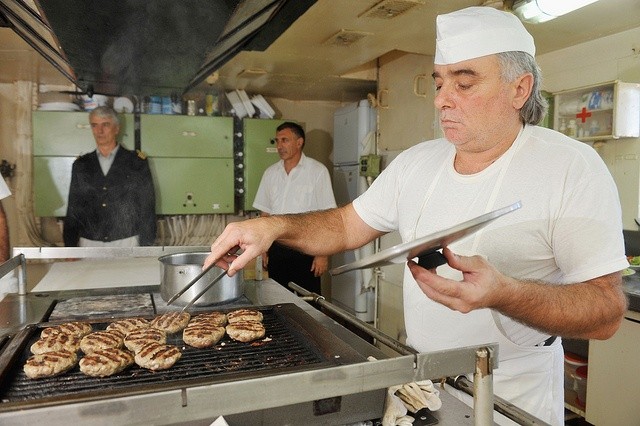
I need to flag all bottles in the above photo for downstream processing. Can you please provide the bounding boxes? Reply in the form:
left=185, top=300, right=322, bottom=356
left=589, top=120, right=598, bottom=136
left=567, top=120, right=577, bottom=137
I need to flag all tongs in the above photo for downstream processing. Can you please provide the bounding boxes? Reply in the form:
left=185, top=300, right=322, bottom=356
left=165, top=244, right=247, bottom=311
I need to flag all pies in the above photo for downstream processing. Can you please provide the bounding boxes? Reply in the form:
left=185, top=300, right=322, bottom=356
left=25, top=350, right=77, bottom=376
left=227, top=308, right=265, bottom=324
left=190, top=312, right=228, bottom=329
left=107, top=318, right=148, bottom=333
left=79, top=332, right=121, bottom=353
left=41, top=322, right=93, bottom=338
left=124, top=329, right=165, bottom=351
left=78, top=349, right=135, bottom=376
left=30, top=333, right=80, bottom=355
left=182, top=324, right=225, bottom=350
left=135, top=343, right=180, bottom=370
left=225, top=320, right=265, bottom=343
left=151, top=311, right=189, bottom=336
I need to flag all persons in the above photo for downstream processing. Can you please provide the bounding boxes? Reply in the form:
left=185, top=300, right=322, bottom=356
left=251, top=122, right=337, bottom=312
left=202, top=5, right=630, bottom=425
left=0, top=172, right=10, bottom=262
left=61, top=107, right=156, bottom=247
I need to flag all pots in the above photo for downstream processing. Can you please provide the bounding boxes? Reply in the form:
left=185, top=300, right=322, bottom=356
left=157, top=249, right=251, bottom=307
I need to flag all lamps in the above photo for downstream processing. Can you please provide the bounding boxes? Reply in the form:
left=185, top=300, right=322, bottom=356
left=512, top=1, right=602, bottom=25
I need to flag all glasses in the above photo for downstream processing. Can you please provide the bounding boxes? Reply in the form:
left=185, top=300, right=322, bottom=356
left=274, top=136, right=297, bottom=143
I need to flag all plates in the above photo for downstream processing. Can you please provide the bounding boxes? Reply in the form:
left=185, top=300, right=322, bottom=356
left=622, top=268, right=635, bottom=278
left=628, top=265, right=640, bottom=271
left=113, top=96, right=134, bottom=115
left=38, top=100, right=79, bottom=113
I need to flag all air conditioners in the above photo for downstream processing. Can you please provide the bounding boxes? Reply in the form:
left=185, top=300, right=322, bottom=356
left=238, top=69, right=278, bottom=80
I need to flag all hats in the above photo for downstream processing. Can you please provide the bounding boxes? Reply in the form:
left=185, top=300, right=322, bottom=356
left=433, top=4, right=536, bottom=65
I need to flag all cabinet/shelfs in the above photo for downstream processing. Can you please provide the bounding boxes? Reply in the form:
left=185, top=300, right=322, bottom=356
left=595, top=138, right=640, bottom=232
left=586, top=309, right=640, bottom=426
left=31, top=111, right=134, bottom=217
left=140, top=114, right=235, bottom=214
left=378, top=230, right=406, bottom=344
left=244, top=119, right=298, bottom=210
left=377, top=52, right=436, bottom=150
left=539, top=54, right=640, bottom=140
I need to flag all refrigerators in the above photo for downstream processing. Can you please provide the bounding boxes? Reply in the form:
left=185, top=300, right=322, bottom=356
left=328, top=99, right=379, bottom=321
